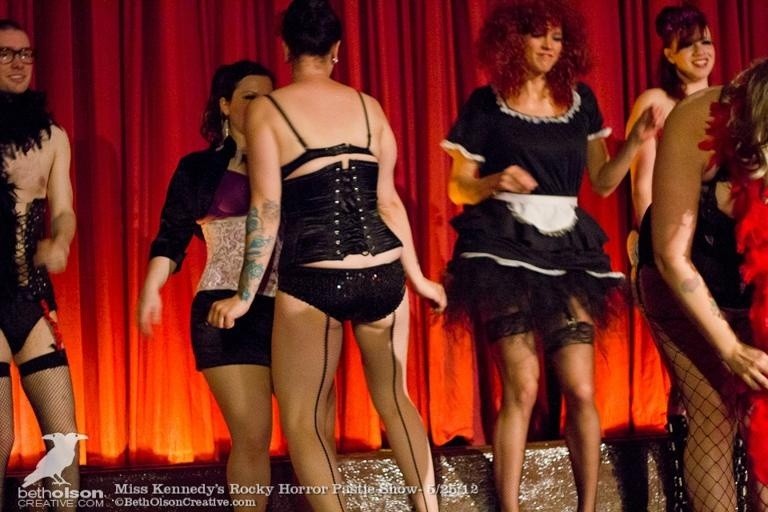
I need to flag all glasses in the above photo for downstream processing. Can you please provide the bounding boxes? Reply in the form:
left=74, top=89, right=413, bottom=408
left=0, top=47, right=35, bottom=64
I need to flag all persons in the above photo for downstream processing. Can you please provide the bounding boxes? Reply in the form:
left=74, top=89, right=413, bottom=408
left=431, top=3, right=662, bottom=511
left=2, top=20, right=81, bottom=510
left=207, top=1, right=449, bottom=512
left=620, top=3, right=756, bottom=509
left=629, top=62, right=767, bottom=511
left=136, top=59, right=277, bottom=510
